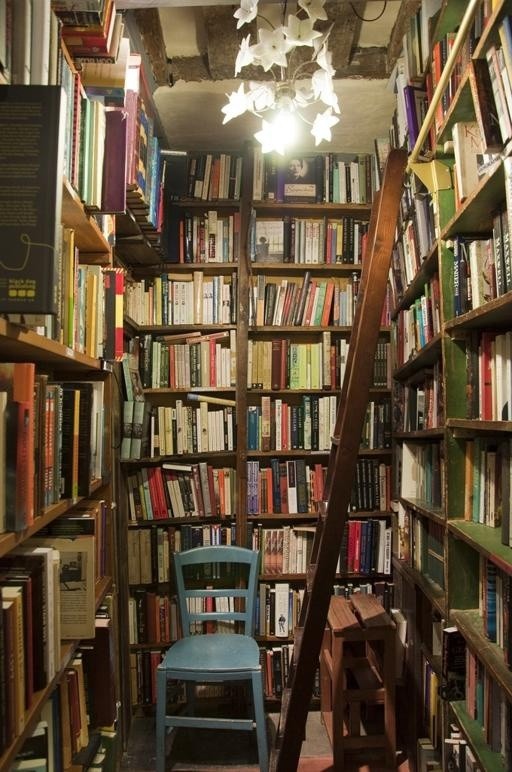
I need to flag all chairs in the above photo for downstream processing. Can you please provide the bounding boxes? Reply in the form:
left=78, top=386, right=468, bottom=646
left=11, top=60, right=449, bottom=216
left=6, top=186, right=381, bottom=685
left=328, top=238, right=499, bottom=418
left=154, top=544, right=268, bottom=772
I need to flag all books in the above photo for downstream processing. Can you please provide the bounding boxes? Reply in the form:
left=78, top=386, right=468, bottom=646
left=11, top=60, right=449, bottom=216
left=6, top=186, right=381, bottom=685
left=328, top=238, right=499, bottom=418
left=2, top=0, right=510, bottom=772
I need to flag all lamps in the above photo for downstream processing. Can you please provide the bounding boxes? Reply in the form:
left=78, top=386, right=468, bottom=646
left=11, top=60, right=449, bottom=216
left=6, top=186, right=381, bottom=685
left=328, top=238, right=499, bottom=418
left=220, top=0, right=344, bottom=154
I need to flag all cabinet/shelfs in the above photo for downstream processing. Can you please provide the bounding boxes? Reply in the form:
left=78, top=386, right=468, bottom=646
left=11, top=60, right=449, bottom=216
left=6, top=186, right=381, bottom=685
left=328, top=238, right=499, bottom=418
left=0, top=0, right=129, bottom=771
left=117, top=141, right=392, bottom=713
left=393, top=0, right=512, bottom=772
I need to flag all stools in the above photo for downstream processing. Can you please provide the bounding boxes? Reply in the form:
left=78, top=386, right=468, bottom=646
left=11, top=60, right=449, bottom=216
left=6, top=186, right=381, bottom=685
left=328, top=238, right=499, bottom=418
left=319, top=593, right=397, bottom=772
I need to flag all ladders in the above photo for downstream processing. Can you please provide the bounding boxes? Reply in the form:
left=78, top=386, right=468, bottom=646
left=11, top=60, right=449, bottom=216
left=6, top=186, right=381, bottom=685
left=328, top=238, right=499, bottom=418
left=271, top=147, right=407, bottom=772
